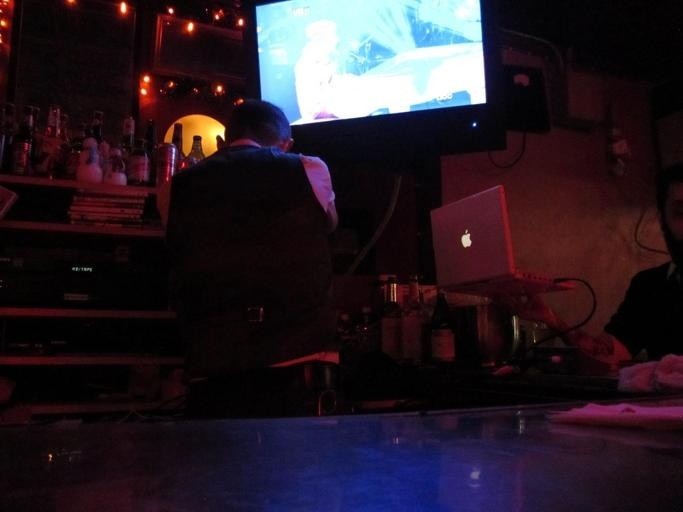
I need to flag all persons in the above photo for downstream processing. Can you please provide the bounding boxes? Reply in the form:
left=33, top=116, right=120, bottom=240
left=495, top=162, right=681, bottom=374
left=166, top=98, right=339, bottom=418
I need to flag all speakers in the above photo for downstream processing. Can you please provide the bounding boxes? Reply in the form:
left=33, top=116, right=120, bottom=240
left=503, top=64, right=550, bottom=133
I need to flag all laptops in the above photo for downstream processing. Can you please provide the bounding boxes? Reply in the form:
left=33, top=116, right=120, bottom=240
left=429, top=184, right=579, bottom=298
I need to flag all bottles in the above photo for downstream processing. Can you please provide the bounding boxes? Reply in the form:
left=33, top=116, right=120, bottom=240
left=15, top=102, right=34, bottom=174
left=355, top=307, right=374, bottom=356
left=32, top=103, right=44, bottom=160
left=186, top=134, right=209, bottom=168
left=0, top=99, right=15, bottom=172
left=59, top=112, right=73, bottom=145
left=105, top=148, right=128, bottom=188
left=167, top=120, right=186, bottom=172
left=63, top=140, right=83, bottom=180
left=75, top=137, right=103, bottom=184
left=90, top=108, right=105, bottom=142
left=145, top=117, right=162, bottom=181
left=433, top=291, right=458, bottom=363
left=403, top=271, right=425, bottom=360
left=118, top=112, right=134, bottom=166
left=338, top=313, right=354, bottom=345
left=379, top=273, right=403, bottom=365
left=40, top=101, right=64, bottom=172
left=128, top=137, right=150, bottom=183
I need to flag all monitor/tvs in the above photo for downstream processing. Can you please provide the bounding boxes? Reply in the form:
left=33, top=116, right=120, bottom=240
left=251, top=1, right=495, bottom=133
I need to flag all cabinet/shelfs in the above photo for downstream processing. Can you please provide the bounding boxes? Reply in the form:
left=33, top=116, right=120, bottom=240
left=0, top=174, right=186, bottom=424
